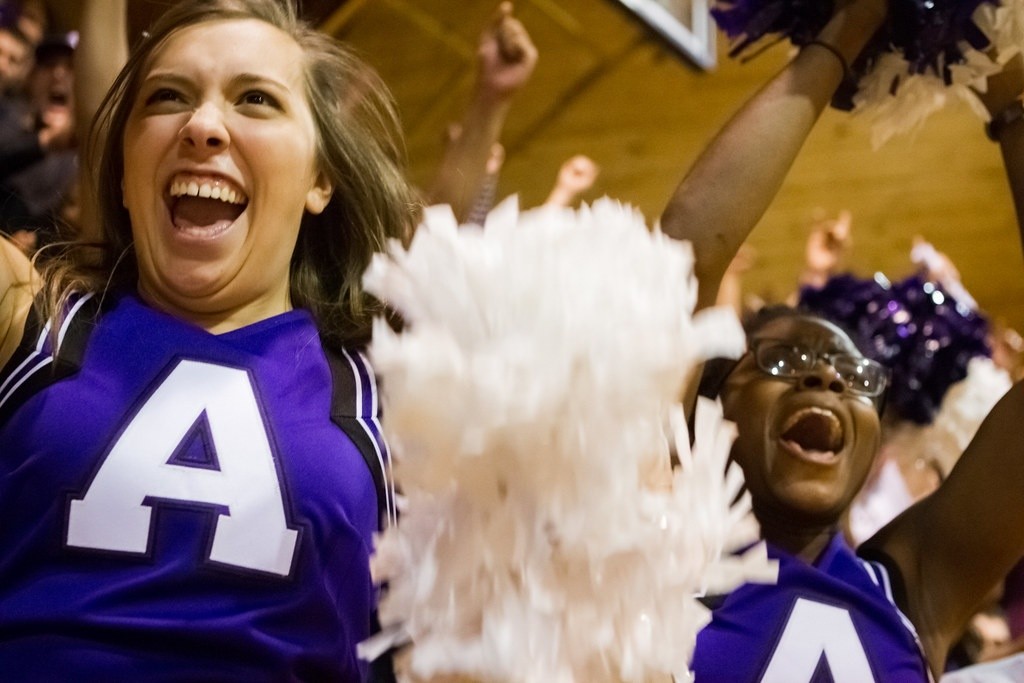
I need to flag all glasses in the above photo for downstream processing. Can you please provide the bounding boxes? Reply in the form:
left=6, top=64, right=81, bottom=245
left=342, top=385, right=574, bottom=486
left=710, top=336, right=892, bottom=419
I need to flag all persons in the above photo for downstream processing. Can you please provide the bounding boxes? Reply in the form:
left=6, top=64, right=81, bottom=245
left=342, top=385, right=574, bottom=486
left=0, top=0, right=1023, bottom=683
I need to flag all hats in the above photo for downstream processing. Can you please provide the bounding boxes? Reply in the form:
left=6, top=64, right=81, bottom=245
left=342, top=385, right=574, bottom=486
left=34, top=30, right=80, bottom=63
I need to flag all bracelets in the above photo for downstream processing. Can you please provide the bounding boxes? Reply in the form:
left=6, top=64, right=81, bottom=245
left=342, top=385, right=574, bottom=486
left=800, top=39, right=847, bottom=88
left=985, top=92, right=1023, bottom=143
